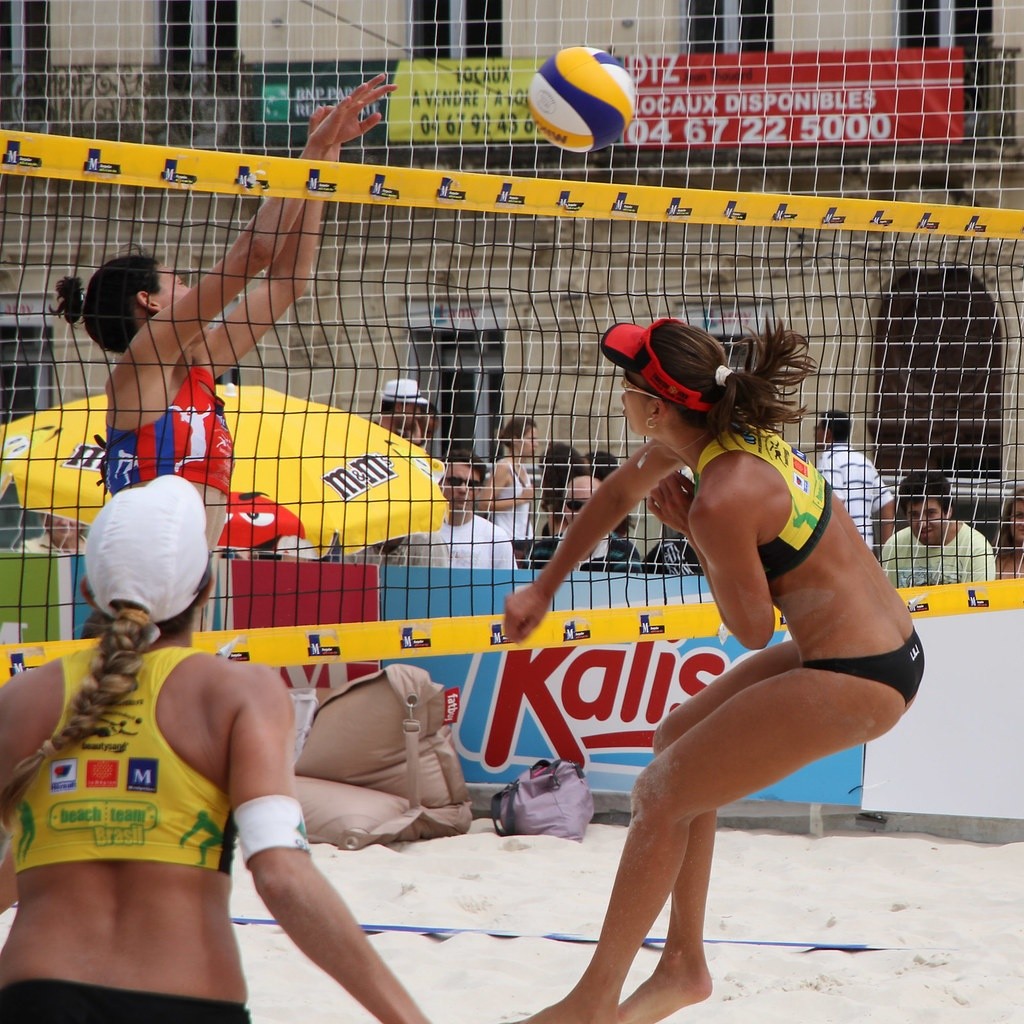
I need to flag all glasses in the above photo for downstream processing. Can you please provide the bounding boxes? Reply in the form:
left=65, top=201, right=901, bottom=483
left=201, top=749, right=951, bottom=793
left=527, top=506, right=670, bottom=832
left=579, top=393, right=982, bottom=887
left=620, top=374, right=662, bottom=400
left=566, top=501, right=583, bottom=511
left=445, top=477, right=480, bottom=487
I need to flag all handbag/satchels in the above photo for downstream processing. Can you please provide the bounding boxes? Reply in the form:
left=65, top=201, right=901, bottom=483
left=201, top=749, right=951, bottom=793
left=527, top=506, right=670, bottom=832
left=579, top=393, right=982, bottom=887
left=490, top=756, right=595, bottom=844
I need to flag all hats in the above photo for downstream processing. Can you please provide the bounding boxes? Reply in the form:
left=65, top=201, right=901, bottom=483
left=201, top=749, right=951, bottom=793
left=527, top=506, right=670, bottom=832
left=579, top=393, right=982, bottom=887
left=380, top=378, right=428, bottom=404
left=600, top=318, right=715, bottom=411
left=83, top=475, right=209, bottom=624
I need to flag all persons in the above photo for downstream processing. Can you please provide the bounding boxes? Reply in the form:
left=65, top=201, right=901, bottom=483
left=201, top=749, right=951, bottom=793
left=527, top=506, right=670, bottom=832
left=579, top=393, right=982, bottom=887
left=23, top=515, right=83, bottom=553
left=1, top=474, right=435, bottom=1024
left=363, top=375, right=1024, bottom=584
left=49, top=72, right=399, bottom=559
left=504, top=318, right=926, bottom=1024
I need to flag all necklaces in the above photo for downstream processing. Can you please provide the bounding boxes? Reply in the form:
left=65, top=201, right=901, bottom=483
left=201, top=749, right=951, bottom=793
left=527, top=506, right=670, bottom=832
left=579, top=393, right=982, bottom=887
left=637, top=433, right=708, bottom=469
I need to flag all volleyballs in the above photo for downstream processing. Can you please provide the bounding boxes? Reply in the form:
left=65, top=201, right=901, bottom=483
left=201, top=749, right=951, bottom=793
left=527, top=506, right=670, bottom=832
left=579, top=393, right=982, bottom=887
left=527, top=48, right=638, bottom=154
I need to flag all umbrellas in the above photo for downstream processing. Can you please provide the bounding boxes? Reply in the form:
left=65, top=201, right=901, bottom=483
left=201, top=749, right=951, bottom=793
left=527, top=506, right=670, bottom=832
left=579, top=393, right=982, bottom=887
left=1, top=383, right=450, bottom=555
